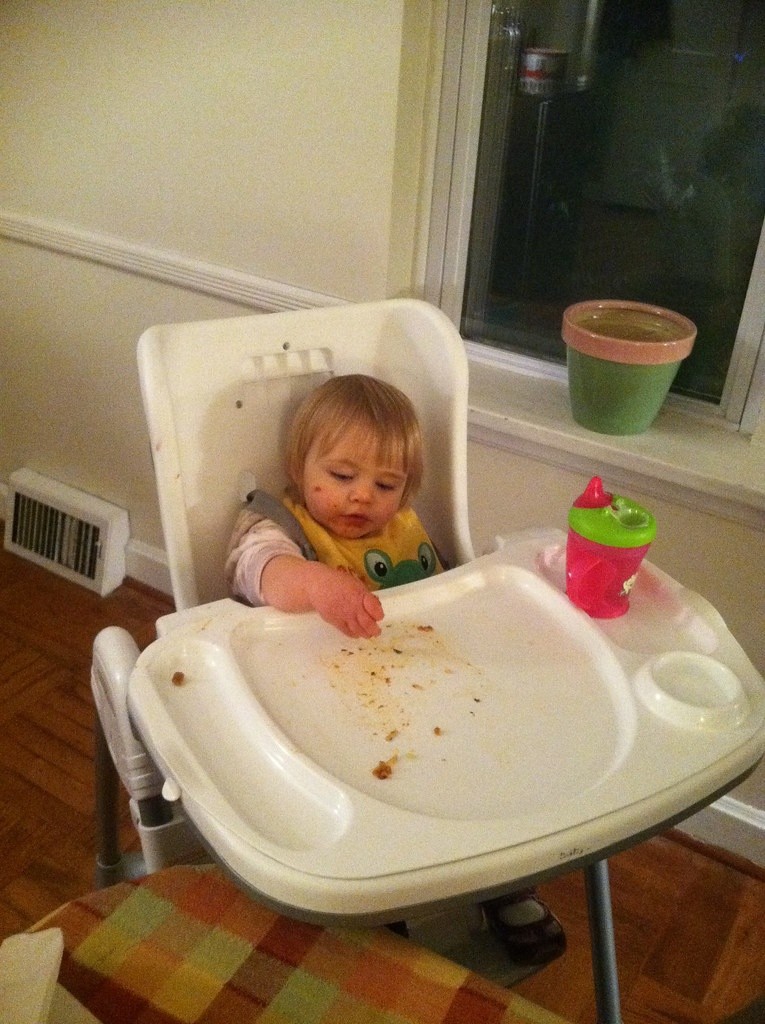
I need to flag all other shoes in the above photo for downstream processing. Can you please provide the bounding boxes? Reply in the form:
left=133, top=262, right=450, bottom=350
left=482, top=887, right=567, bottom=965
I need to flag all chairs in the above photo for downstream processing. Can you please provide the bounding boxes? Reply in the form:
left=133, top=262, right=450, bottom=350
left=91, top=297, right=764, bottom=1024
left=1, top=865, right=582, bottom=1024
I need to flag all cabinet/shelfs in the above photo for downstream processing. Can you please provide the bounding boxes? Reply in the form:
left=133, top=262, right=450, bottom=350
left=491, top=87, right=608, bottom=305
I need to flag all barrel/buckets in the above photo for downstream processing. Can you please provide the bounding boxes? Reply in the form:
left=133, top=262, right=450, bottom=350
left=562, top=299, right=698, bottom=435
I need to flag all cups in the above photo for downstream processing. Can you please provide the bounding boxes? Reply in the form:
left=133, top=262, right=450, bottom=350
left=565, top=475, right=657, bottom=619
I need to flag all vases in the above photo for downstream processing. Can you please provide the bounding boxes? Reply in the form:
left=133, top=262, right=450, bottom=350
left=561, top=299, right=697, bottom=436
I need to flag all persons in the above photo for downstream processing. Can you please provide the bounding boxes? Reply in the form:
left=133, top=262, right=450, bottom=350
left=224, top=374, right=566, bottom=960
